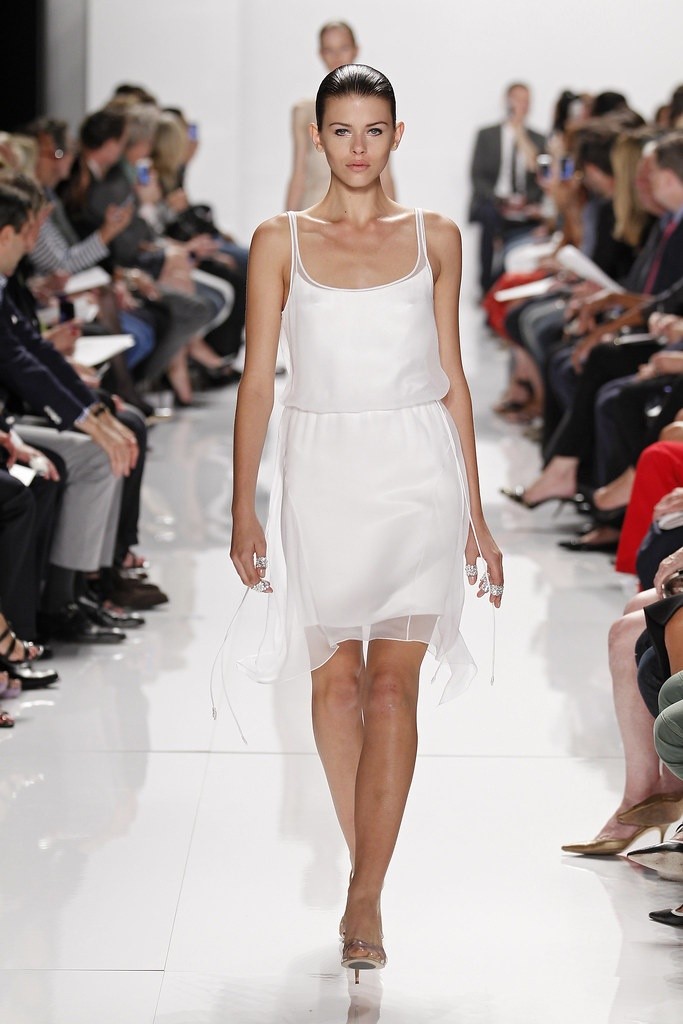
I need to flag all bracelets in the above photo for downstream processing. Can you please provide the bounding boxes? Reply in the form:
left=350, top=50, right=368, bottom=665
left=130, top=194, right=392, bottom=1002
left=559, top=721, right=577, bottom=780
left=93, top=403, right=110, bottom=417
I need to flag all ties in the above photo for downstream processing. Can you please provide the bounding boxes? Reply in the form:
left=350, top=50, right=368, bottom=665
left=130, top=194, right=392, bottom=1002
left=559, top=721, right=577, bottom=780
left=511, top=138, right=521, bottom=194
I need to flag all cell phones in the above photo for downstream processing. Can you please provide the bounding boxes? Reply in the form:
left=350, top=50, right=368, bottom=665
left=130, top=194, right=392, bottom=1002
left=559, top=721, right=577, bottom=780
left=8, top=462, right=39, bottom=488
left=136, top=157, right=151, bottom=184
left=187, top=125, right=196, bottom=140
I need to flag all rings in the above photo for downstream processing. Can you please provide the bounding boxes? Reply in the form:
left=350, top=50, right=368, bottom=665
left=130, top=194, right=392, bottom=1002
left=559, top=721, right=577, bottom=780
left=251, top=585, right=263, bottom=592
left=479, top=577, right=490, bottom=593
left=480, top=573, right=486, bottom=581
left=491, top=584, right=504, bottom=596
left=255, top=580, right=270, bottom=592
left=256, top=556, right=268, bottom=568
left=465, top=565, right=477, bottom=576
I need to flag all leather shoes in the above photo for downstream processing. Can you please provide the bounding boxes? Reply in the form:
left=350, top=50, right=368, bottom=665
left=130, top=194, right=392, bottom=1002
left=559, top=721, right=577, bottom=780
left=41, top=602, right=126, bottom=643
left=0, top=657, right=58, bottom=691
left=627, top=840, right=683, bottom=885
left=649, top=904, right=683, bottom=925
left=69, top=596, right=144, bottom=628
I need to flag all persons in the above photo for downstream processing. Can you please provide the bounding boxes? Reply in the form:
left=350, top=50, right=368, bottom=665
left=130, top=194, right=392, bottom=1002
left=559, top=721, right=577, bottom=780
left=285, top=19, right=398, bottom=216
left=231, top=65, right=505, bottom=987
left=472, top=82, right=548, bottom=302
left=475, top=85, right=682, bottom=927
left=0, top=83, right=289, bottom=728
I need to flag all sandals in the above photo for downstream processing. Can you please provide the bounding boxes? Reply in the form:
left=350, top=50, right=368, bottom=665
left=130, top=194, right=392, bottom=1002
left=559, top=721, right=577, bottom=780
left=0, top=614, right=45, bottom=665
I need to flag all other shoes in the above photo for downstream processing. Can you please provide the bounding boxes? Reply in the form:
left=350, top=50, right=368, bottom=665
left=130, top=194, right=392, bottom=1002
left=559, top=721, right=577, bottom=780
left=572, top=479, right=627, bottom=525
left=191, top=359, right=244, bottom=390
left=101, top=571, right=169, bottom=608
left=0, top=708, right=14, bottom=728
left=559, top=531, right=619, bottom=554
left=490, top=371, right=544, bottom=442
left=120, top=552, right=145, bottom=567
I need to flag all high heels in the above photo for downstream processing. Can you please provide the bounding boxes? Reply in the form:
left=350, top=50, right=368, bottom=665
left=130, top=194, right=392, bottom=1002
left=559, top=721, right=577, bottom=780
left=339, top=867, right=383, bottom=943
left=498, top=485, right=575, bottom=519
left=617, top=787, right=683, bottom=825
left=341, top=939, right=387, bottom=983
left=152, top=372, right=208, bottom=409
left=561, top=822, right=670, bottom=856
